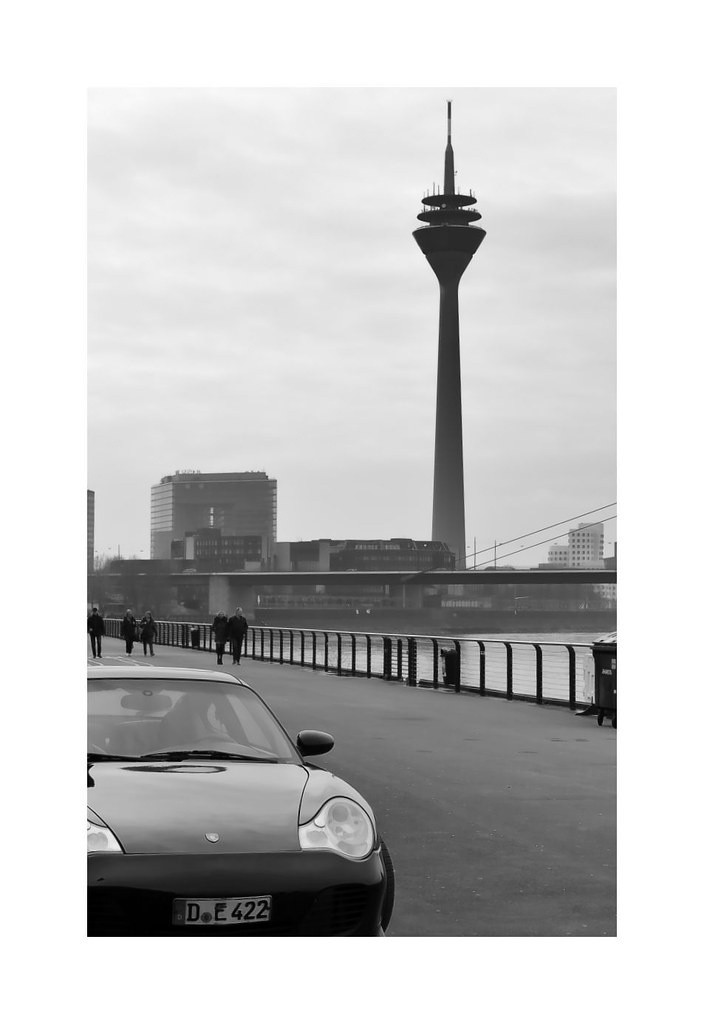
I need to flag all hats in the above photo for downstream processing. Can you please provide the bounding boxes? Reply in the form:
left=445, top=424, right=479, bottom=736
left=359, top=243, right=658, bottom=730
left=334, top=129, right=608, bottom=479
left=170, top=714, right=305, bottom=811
left=92, top=608, right=97, bottom=612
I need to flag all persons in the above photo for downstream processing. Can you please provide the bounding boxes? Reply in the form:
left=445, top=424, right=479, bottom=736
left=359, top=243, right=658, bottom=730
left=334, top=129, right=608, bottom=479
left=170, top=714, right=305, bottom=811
left=140, top=611, right=157, bottom=656
left=87, top=608, right=106, bottom=658
left=211, top=610, right=229, bottom=665
left=120, top=609, right=139, bottom=657
left=229, top=607, right=248, bottom=665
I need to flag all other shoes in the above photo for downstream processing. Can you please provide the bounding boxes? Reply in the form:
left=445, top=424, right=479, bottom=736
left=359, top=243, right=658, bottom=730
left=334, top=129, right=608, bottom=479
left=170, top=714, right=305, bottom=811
left=217, top=659, right=223, bottom=665
left=150, top=653, right=155, bottom=657
left=93, top=655, right=96, bottom=658
left=97, top=654, right=102, bottom=658
left=232, top=660, right=236, bottom=664
left=237, top=661, right=240, bottom=665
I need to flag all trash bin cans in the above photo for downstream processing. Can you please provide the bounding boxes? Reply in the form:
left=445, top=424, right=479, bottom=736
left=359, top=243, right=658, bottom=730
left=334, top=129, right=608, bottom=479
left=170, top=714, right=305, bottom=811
left=440, top=647, right=458, bottom=684
left=589, top=633, right=617, bottom=730
left=191, top=628, right=200, bottom=646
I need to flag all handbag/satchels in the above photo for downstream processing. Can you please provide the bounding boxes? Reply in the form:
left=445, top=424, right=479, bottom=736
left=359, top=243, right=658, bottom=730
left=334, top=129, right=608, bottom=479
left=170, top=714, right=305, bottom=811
left=138, top=629, right=143, bottom=641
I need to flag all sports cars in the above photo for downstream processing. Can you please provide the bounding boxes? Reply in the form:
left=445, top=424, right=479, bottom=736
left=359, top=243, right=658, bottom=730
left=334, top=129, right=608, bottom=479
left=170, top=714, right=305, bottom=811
left=86, top=665, right=396, bottom=937
